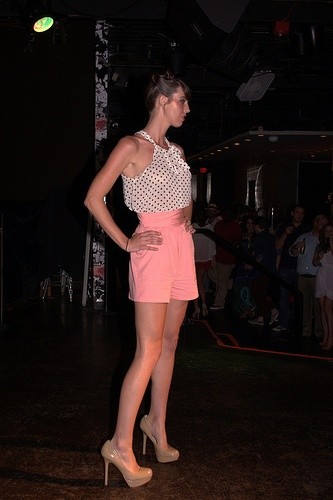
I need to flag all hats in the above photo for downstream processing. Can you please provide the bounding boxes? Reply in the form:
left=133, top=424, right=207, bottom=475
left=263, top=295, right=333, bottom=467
left=204, top=204, right=220, bottom=214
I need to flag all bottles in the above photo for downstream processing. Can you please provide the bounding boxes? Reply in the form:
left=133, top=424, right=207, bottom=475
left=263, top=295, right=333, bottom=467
left=322, top=237, right=330, bottom=252
left=300, top=236, right=305, bottom=254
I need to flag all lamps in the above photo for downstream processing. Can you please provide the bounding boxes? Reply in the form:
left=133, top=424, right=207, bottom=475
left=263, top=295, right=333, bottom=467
left=24, top=0, right=54, bottom=33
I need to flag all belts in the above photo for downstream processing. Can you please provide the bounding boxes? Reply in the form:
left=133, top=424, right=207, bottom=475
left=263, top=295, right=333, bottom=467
left=301, top=274, right=316, bottom=278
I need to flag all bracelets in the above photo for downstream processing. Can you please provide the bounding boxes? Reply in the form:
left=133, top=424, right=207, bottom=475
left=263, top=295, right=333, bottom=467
left=125, top=239, right=129, bottom=251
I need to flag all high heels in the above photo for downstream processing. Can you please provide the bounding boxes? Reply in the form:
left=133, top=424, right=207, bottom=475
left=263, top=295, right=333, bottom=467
left=101, top=440, right=152, bottom=487
left=193, top=308, right=200, bottom=319
left=202, top=303, right=208, bottom=316
left=140, top=415, right=179, bottom=463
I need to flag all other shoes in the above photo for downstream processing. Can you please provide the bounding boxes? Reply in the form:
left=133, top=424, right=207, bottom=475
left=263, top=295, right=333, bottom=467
left=272, top=325, right=287, bottom=331
left=321, top=342, right=333, bottom=351
left=303, top=327, right=311, bottom=336
left=248, top=315, right=265, bottom=326
left=210, top=303, right=224, bottom=310
left=268, top=308, right=279, bottom=325
left=319, top=340, right=327, bottom=345
left=315, top=331, right=323, bottom=338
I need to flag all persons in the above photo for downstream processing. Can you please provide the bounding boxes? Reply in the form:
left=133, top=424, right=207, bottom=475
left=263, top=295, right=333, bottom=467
left=289, top=215, right=327, bottom=337
left=313, top=223, right=333, bottom=350
left=83, top=73, right=199, bottom=489
left=273, top=205, right=307, bottom=331
left=192, top=202, right=279, bottom=331
